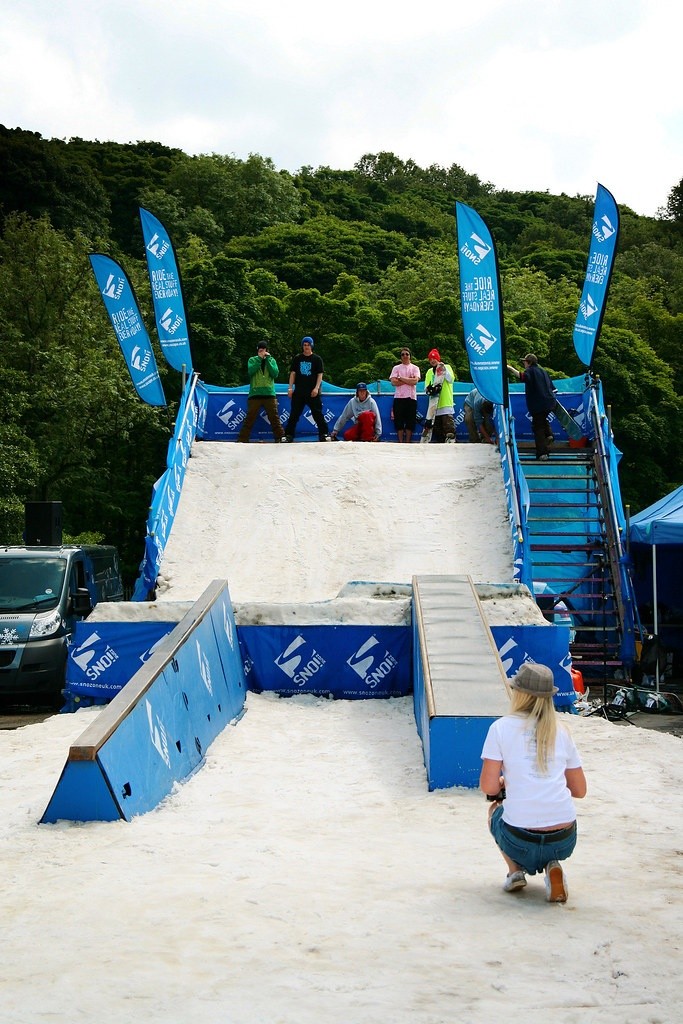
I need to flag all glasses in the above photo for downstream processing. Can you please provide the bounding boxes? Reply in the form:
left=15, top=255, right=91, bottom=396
left=401, top=353, right=410, bottom=356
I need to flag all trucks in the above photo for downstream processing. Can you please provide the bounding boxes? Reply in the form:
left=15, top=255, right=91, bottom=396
left=0, top=544, right=125, bottom=695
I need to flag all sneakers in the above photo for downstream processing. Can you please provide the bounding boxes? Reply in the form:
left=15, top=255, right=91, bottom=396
left=503, top=871, right=526, bottom=891
left=543, top=860, right=568, bottom=902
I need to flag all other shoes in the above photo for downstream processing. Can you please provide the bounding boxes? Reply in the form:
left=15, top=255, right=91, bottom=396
left=546, top=436, right=553, bottom=450
left=538, top=454, right=549, bottom=461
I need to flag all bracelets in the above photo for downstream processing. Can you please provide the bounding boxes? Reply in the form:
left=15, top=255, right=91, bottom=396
left=398, top=377, right=401, bottom=378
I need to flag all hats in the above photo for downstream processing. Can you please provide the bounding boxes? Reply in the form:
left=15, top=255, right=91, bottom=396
left=356, top=382, right=367, bottom=390
left=301, top=337, right=314, bottom=348
left=428, top=349, right=440, bottom=361
left=520, top=354, right=537, bottom=362
left=507, top=663, right=559, bottom=696
left=256, top=341, right=267, bottom=350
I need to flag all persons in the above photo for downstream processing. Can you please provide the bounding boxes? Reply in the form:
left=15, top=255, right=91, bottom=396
left=282, top=337, right=331, bottom=441
left=464, top=388, right=493, bottom=443
left=507, top=354, right=557, bottom=461
left=479, top=663, right=587, bottom=903
left=425, top=349, right=456, bottom=442
left=389, top=348, right=421, bottom=442
left=238, top=340, right=284, bottom=443
left=330, top=382, right=382, bottom=442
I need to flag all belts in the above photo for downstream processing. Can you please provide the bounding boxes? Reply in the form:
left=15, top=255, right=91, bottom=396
left=504, top=823, right=575, bottom=843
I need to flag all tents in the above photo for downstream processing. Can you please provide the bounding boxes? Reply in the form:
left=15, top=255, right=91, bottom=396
left=627, top=483, right=683, bottom=694
